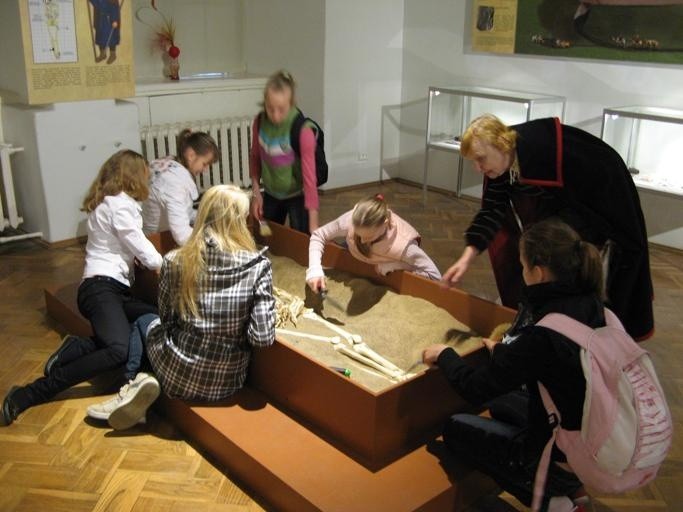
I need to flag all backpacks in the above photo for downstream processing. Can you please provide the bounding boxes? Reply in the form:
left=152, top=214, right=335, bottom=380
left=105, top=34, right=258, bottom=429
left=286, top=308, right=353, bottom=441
left=532, top=306, right=674, bottom=502
left=257, top=108, right=328, bottom=187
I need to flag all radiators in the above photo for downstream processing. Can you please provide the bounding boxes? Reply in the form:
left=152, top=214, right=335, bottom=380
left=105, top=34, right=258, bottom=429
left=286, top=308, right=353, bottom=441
left=139, top=116, right=253, bottom=194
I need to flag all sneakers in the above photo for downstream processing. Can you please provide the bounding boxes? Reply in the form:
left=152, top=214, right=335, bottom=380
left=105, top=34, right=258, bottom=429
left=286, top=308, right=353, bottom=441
left=86, top=384, right=132, bottom=420
left=107, top=374, right=161, bottom=432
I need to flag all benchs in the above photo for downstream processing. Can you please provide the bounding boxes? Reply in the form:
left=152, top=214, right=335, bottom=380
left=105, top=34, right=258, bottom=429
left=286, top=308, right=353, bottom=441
left=44, top=282, right=492, bottom=511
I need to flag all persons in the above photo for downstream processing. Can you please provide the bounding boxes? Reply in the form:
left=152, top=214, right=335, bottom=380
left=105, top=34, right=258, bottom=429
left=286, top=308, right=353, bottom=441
left=87, top=185, right=276, bottom=432
left=2, top=150, right=164, bottom=427
left=91, top=0, right=121, bottom=64
left=442, top=114, right=656, bottom=342
left=249, top=69, right=321, bottom=235
left=144, top=128, right=221, bottom=247
left=304, top=193, right=442, bottom=295
left=44, top=0, right=59, bottom=58
left=421, top=223, right=605, bottom=512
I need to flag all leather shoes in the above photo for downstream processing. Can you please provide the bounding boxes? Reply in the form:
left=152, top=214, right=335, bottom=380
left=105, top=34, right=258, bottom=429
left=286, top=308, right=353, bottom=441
left=43, top=333, right=79, bottom=378
left=2, top=384, right=24, bottom=425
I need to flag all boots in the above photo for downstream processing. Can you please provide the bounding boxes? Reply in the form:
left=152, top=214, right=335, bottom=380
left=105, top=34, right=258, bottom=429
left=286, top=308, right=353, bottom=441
left=94, top=48, right=118, bottom=64
